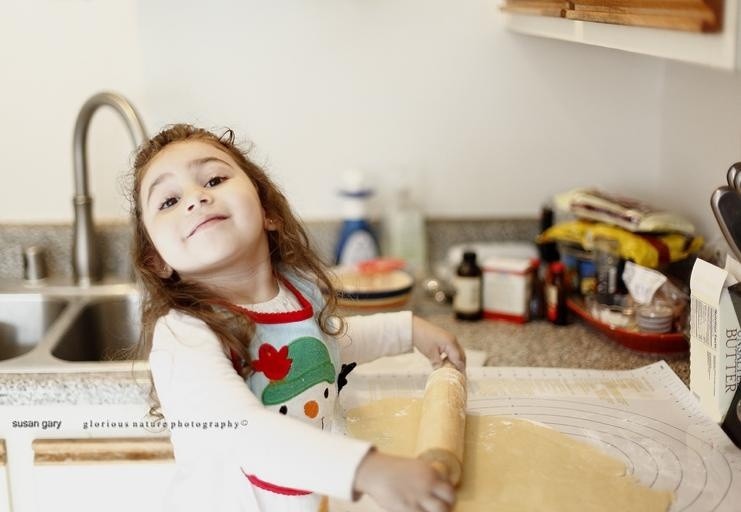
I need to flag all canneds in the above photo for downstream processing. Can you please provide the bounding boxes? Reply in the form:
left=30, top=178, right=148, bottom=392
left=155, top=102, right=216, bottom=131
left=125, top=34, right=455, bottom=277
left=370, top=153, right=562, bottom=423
left=636, top=302, right=673, bottom=334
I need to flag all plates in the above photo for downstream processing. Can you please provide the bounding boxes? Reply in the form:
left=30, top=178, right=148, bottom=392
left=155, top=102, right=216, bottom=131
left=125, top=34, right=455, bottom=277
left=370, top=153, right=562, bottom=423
left=560, top=297, right=690, bottom=357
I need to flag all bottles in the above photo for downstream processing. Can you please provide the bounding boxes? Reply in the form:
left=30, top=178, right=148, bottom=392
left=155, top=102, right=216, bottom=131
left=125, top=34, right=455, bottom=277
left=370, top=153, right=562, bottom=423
left=546, top=262, right=567, bottom=324
left=380, top=186, right=427, bottom=280
left=536, top=207, right=558, bottom=317
left=453, top=251, right=485, bottom=322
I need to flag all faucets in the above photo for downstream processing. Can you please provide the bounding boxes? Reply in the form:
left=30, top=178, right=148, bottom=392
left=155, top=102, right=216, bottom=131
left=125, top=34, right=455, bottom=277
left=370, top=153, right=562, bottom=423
left=70, top=89, right=149, bottom=285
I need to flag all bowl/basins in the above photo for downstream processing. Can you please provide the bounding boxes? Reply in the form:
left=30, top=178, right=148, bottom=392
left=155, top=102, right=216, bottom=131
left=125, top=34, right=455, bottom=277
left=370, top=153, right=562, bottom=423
left=319, top=266, right=413, bottom=312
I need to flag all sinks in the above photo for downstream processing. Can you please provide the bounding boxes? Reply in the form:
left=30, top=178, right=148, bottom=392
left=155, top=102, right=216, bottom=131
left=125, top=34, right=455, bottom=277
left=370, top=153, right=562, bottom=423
left=56, top=276, right=154, bottom=372
left=0, top=276, right=66, bottom=362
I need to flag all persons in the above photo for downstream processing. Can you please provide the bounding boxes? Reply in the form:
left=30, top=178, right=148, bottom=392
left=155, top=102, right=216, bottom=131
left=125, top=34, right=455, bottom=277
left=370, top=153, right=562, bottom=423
left=123, top=123, right=466, bottom=512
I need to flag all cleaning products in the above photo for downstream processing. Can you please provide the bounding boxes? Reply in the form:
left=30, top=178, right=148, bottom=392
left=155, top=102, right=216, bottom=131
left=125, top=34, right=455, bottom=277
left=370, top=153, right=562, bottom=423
left=332, top=176, right=382, bottom=265
